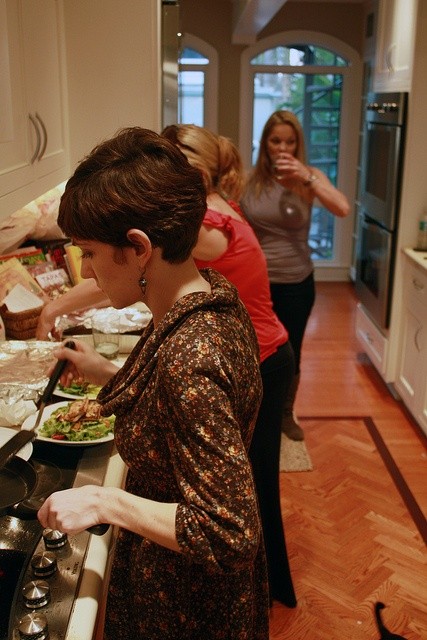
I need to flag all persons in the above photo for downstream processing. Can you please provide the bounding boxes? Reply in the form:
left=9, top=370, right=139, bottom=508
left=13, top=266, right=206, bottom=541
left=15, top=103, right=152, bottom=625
left=237, top=110, right=350, bottom=443
left=36, top=127, right=268, bottom=639
left=38, top=123, right=298, bottom=609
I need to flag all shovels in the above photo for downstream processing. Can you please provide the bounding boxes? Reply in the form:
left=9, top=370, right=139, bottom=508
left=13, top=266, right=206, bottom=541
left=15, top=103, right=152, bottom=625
left=0, top=340, right=75, bottom=471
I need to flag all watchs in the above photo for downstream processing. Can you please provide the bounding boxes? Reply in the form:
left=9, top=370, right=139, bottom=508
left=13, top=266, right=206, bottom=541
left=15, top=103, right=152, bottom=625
left=303, top=169, right=317, bottom=186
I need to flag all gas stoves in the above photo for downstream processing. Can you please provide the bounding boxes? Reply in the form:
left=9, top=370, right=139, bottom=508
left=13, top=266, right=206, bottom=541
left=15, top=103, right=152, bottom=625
left=0, top=440, right=115, bottom=640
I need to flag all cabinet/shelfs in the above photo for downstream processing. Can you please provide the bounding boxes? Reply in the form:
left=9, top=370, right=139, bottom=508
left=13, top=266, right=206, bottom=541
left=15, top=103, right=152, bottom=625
left=374, top=0, right=417, bottom=94
left=1, top=16, right=71, bottom=224
left=394, top=250, right=427, bottom=434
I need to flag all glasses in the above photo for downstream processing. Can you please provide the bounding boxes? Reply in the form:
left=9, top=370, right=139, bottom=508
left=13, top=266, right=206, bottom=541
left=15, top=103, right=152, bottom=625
left=161, top=125, right=195, bottom=152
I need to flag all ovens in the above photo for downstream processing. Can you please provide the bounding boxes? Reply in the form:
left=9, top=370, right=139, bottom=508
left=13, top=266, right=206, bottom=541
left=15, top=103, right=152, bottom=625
left=347, top=91, right=408, bottom=329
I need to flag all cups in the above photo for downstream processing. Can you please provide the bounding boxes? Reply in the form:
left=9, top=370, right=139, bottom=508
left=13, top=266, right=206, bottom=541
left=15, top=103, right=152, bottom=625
left=90, top=312, right=120, bottom=360
left=273, top=154, right=286, bottom=177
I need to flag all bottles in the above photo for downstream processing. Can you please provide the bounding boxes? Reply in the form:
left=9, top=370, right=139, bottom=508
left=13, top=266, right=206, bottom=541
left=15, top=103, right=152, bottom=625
left=417, top=211, right=427, bottom=252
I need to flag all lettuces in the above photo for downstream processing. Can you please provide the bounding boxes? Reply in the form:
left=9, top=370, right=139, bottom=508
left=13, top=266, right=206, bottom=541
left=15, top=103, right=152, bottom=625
left=40, top=407, right=116, bottom=440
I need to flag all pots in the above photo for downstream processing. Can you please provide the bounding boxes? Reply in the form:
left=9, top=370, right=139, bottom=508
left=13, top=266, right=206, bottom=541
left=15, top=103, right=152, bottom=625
left=0, top=456, right=110, bottom=535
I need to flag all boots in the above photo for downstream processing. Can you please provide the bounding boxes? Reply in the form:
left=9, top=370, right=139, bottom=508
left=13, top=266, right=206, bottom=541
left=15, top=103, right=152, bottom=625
left=281, top=372, right=304, bottom=442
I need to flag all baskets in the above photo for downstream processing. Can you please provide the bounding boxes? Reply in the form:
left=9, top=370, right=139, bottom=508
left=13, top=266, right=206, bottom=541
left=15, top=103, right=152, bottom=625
left=0, top=305, right=45, bottom=340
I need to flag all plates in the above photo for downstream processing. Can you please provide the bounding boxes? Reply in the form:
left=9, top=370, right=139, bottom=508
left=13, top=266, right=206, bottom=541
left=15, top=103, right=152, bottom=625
left=21, top=401, right=115, bottom=447
left=52, top=383, right=97, bottom=400
left=0, top=427, right=33, bottom=462
